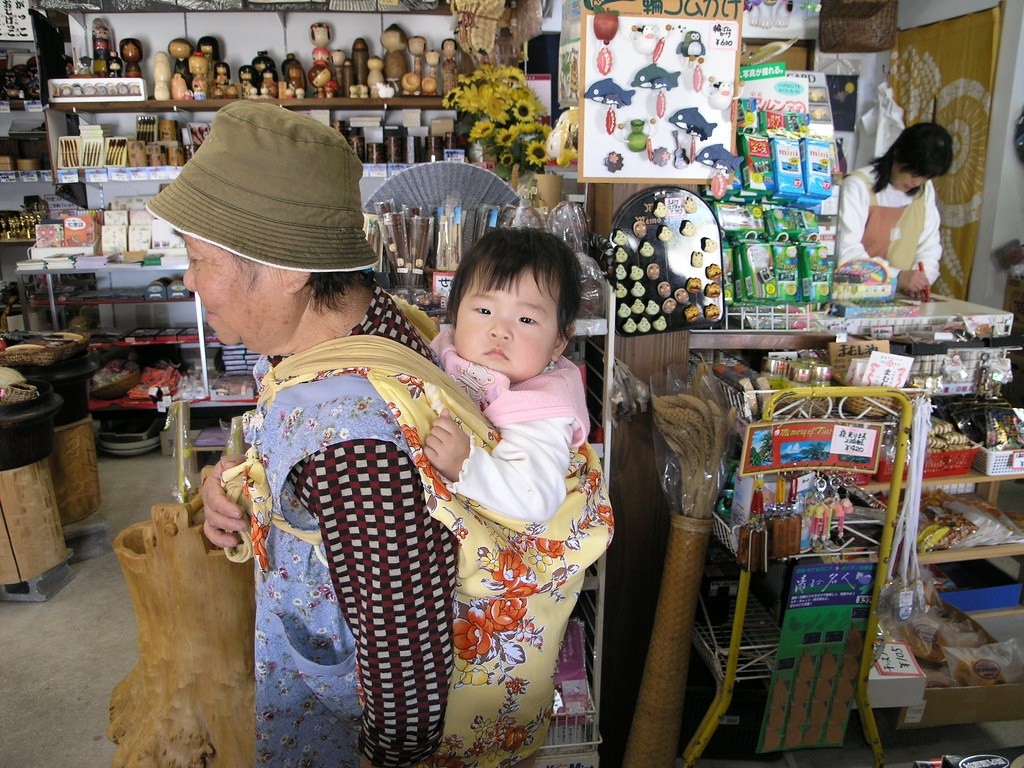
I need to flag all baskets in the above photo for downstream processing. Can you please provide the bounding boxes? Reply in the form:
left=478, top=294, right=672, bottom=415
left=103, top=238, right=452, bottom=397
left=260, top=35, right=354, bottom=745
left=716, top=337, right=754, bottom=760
left=965, top=435, right=1024, bottom=476
left=819, top=468, right=871, bottom=488
left=0, top=328, right=92, bottom=366
left=875, top=446, right=980, bottom=482
left=842, top=393, right=918, bottom=419
left=757, top=387, right=835, bottom=415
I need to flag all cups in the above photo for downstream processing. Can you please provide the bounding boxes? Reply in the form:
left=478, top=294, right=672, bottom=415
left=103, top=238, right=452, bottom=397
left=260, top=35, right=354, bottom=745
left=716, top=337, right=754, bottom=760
left=127, top=118, right=470, bottom=166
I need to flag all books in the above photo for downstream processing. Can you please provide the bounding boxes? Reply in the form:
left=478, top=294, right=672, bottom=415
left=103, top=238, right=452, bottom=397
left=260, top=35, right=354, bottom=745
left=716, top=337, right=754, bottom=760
left=222, top=345, right=261, bottom=376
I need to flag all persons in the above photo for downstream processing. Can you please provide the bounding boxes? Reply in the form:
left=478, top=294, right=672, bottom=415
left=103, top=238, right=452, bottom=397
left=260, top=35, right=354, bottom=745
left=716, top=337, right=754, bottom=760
left=836, top=123, right=954, bottom=299
left=62, top=17, right=459, bottom=99
left=424, top=226, right=614, bottom=768
left=144, top=99, right=461, bottom=768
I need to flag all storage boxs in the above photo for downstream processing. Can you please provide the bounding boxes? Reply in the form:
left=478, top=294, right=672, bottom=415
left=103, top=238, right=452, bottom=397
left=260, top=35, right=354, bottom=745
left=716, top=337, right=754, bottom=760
left=874, top=599, right=1024, bottom=729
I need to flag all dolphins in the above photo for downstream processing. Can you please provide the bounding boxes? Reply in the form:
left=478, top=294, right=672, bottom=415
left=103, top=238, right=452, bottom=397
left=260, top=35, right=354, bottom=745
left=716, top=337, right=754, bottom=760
left=668, top=106, right=718, bottom=141
left=697, top=143, right=745, bottom=175
left=583, top=76, right=635, bottom=109
left=630, top=62, right=680, bottom=91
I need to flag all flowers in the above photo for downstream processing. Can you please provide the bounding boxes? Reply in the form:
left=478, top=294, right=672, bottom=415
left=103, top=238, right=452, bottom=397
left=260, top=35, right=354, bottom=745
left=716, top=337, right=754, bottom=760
left=440, top=60, right=576, bottom=182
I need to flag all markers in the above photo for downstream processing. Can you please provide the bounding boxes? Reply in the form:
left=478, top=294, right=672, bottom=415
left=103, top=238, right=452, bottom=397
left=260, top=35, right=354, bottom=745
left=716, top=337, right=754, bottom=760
left=918, top=262, right=930, bottom=300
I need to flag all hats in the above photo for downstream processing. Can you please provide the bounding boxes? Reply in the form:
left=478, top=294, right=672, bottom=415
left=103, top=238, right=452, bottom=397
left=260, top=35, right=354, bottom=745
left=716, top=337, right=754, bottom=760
left=144, top=100, right=379, bottom=273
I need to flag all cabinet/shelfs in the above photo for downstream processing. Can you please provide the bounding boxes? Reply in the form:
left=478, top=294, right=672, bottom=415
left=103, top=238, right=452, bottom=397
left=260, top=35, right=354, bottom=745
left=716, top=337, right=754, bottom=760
left=853, top=468, right=1024, bottom=617
left=0, top=0, right=529, bottom=410
left=683, top=383, right=910, bottom=768
